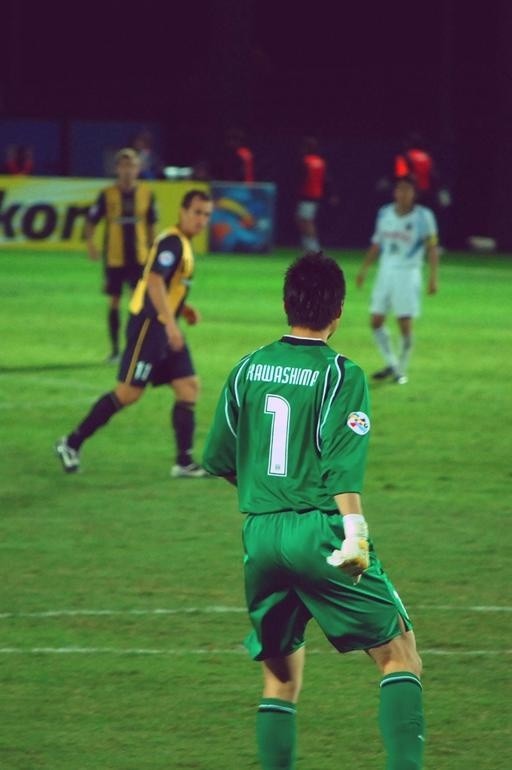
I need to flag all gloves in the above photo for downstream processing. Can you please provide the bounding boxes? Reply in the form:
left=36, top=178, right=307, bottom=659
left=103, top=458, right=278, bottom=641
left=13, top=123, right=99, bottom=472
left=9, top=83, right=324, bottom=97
left=326, top=514, right=370, bottom=586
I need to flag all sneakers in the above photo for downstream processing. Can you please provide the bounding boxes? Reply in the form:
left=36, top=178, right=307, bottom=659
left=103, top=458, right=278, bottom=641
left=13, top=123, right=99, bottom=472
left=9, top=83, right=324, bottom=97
left=55, top=437, right=80, bottom=472
left=170, top=463, right=210, bottom=478
left=373, top=365, right=407, bottom=384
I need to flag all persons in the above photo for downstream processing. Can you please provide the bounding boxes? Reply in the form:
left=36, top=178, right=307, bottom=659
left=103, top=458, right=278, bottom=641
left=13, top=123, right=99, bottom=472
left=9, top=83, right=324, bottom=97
left=3, top=126, right=431, bottom=252
left=54, top=188, right=213, bottom=479
left=355, top=176, right=438, bottom=383
left=82, top=148, right=158, bottom=364
left=199, top=249, right=425, bottom=770
